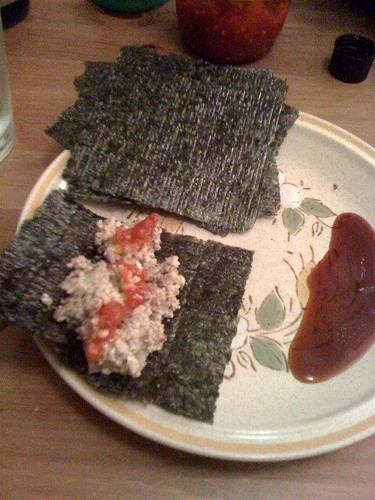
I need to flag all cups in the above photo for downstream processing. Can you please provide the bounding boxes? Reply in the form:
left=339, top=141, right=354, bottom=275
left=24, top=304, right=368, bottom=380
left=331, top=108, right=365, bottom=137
left=176, top=0, right=290, bottom=65
left=0, top=14, right=15, bottom=163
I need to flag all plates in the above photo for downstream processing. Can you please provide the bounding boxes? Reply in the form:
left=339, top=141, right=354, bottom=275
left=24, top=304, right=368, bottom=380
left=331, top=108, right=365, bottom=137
left=16, top=111, right=375, bottom=463
